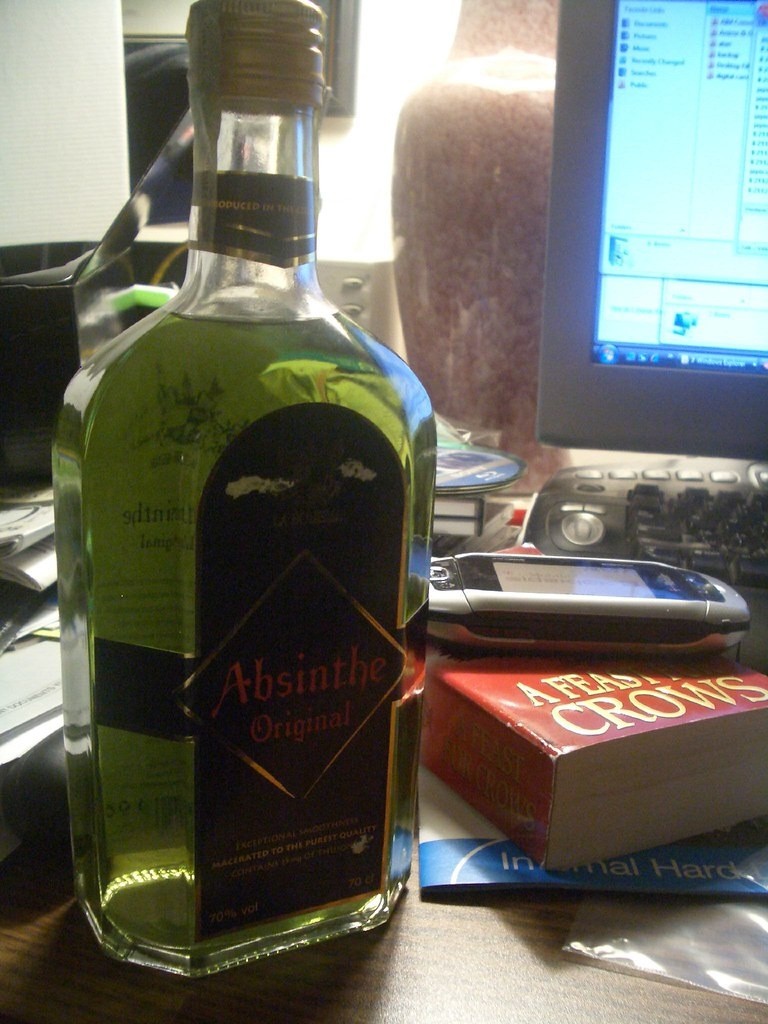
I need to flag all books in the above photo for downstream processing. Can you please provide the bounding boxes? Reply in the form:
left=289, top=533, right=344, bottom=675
left=416, top=765, right=768, bottom=893
left=421, top=639, right=768, bottom=870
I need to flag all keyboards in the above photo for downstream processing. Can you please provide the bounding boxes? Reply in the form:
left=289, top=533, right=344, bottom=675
left=522, top=467, right=768, bottom=675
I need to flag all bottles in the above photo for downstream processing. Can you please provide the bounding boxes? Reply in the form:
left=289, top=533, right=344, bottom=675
left=50, top=1, right=436, bottom=979
left=391, top=0, right=574, bottom=497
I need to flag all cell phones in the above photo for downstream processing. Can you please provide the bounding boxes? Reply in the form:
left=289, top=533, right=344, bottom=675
left=425, top=551, right=749, bottom=657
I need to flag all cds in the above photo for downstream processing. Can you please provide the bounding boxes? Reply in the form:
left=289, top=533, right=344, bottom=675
left=432, top=445, right=528, bottom=496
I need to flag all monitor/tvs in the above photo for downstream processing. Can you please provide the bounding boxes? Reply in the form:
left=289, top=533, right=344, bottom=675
left=537, top=0, right=768, bottom=464
